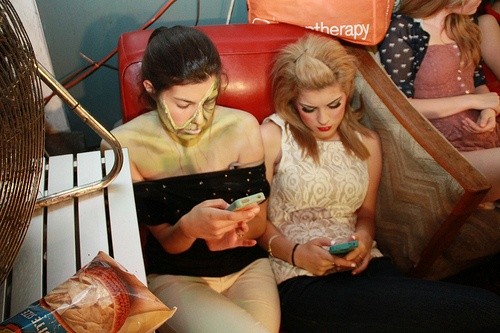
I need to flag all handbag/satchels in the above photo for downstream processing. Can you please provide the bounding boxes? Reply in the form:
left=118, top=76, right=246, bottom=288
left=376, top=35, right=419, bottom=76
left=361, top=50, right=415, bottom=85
left=247, top=0, right=394, bottom=46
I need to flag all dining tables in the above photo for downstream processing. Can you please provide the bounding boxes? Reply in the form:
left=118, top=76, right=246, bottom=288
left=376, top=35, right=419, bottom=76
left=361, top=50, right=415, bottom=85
left=0, top=147, right=155, bottom=333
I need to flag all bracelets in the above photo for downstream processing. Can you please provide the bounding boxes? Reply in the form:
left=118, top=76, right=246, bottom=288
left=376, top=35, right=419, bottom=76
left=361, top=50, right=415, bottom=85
left=291, top=244, right=300, bottom=266
left=267, top=235, right=283, bottom=257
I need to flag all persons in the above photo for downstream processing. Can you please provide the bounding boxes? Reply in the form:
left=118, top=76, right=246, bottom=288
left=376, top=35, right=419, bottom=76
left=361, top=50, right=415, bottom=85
left=378, top=0, right=499, bottom=203
left=258, top=34, right=499, bottom=332
left=98, top=25, right=281, bottom=333
left=474, top=0, right=499, bottom=97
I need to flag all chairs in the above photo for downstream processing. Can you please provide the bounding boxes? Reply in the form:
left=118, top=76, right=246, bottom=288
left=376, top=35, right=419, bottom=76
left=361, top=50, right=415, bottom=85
left=346, top=0, right=500, bottom=280
left=118, top=20, right=353, bottom=125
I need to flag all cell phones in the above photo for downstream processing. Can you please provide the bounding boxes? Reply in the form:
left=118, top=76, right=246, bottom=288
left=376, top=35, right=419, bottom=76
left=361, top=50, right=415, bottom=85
left=329, top=240, right=359, bottom=256
left=226, top=192, right=266, bottom=212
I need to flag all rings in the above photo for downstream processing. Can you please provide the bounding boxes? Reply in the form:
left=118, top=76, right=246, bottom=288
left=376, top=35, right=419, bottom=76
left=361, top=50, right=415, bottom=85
left=236, top=231, right=244, bottom=239
left=359, top=255, right=363, bottom=260
left=333, top=263, right=335, bottom=265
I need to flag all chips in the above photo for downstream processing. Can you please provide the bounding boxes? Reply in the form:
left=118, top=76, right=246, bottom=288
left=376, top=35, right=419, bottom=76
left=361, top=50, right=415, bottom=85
left=40, top=274, right=114, bottom=333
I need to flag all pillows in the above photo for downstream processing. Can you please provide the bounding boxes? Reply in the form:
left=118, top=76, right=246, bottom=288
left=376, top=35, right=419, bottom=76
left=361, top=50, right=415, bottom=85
left=246, top=0, right=395, bottom=45
